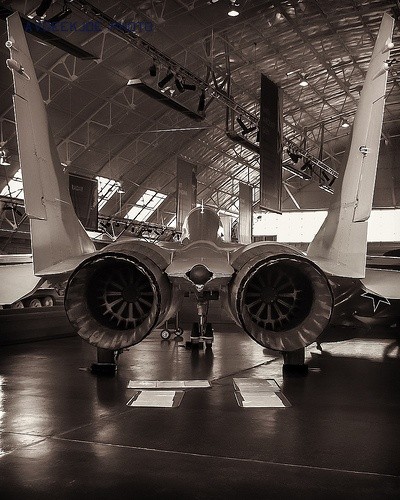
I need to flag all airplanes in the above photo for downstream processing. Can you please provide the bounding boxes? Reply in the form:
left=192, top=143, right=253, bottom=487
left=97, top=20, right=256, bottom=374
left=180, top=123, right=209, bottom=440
left=0, top=11, right=400, bottom=381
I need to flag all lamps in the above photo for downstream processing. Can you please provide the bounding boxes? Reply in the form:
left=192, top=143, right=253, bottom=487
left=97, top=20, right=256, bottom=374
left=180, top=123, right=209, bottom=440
left=150, top=57, right=336, bottom=186
